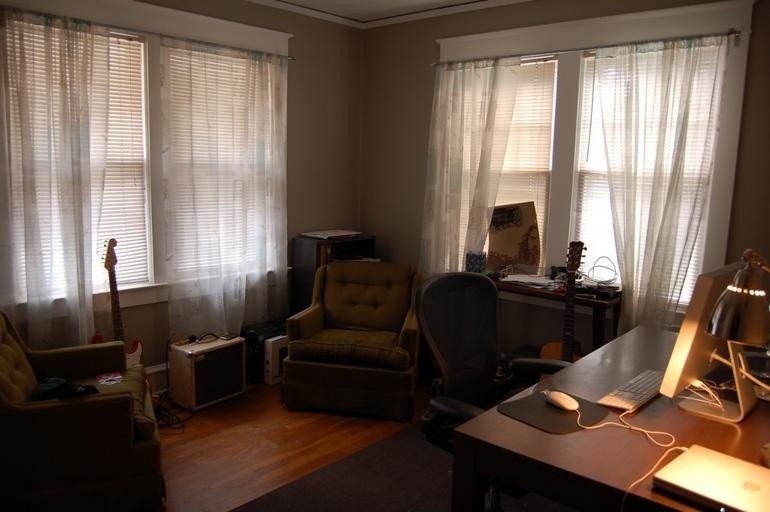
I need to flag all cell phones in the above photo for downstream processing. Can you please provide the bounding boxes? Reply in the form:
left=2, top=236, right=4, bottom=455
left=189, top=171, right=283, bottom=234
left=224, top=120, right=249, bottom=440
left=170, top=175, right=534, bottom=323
left=220, top=334, right=237, bottom=339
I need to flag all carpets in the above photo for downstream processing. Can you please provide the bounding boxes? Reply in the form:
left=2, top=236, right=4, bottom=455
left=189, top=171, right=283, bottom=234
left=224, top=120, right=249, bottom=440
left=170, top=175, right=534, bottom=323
left=219, top=421, right=578, bottom=512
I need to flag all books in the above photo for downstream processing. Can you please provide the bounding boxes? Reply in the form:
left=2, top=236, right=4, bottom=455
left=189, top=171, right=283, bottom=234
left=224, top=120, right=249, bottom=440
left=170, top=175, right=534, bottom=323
left=505, top=275, right=555, bottom=291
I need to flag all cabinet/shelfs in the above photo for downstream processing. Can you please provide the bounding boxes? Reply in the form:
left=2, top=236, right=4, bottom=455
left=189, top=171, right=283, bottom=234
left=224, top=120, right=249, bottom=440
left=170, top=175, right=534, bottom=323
left=289, top=232, right=377, bottom=314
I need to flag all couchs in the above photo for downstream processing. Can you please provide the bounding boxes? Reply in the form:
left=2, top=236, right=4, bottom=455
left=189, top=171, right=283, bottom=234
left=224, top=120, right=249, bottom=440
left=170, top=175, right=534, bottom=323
left=279, top=259, right=425, bottom=419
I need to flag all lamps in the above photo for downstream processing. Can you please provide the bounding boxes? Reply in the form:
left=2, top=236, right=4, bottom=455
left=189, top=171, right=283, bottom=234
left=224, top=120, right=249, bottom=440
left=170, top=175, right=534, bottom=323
left=726, top=249, right=769, bottom=298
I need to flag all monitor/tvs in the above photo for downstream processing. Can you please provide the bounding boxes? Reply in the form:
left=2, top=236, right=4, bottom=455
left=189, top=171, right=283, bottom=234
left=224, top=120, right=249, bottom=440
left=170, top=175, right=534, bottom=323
left=659, top=261, right=757, bottom=424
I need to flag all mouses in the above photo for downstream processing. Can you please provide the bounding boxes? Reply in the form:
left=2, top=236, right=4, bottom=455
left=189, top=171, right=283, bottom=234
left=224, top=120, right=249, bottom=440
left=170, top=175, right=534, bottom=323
left=546, top=391, right=579, bottom=411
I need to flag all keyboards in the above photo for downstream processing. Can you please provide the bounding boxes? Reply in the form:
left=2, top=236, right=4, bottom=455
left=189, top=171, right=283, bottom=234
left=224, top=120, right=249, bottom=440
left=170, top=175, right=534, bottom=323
left=596, top=369, right=665, bottom=413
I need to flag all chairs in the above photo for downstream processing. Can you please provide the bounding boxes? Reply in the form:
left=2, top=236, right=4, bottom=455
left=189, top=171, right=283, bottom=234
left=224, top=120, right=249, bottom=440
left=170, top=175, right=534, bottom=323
left=1, top=309, right=167, bottom=510
left=414, top=271, right=573, bottom=512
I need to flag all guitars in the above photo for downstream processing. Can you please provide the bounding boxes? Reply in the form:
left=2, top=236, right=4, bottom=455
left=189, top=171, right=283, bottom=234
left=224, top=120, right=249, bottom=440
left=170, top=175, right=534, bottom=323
left=91, top=239, right=159, bottom=410
left=540, top=242, right=587, bottom=383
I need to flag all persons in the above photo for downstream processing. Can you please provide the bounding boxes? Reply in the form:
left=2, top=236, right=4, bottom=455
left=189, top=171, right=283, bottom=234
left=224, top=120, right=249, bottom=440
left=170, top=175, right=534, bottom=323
left=516, top=225, right=539, bottom=265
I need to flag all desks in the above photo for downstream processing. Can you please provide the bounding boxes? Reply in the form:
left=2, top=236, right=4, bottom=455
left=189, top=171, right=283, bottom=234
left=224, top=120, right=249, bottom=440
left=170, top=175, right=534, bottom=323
left=483, top=265, right=625, bottom=379
left=448, top=323, right=769, bottom=512
